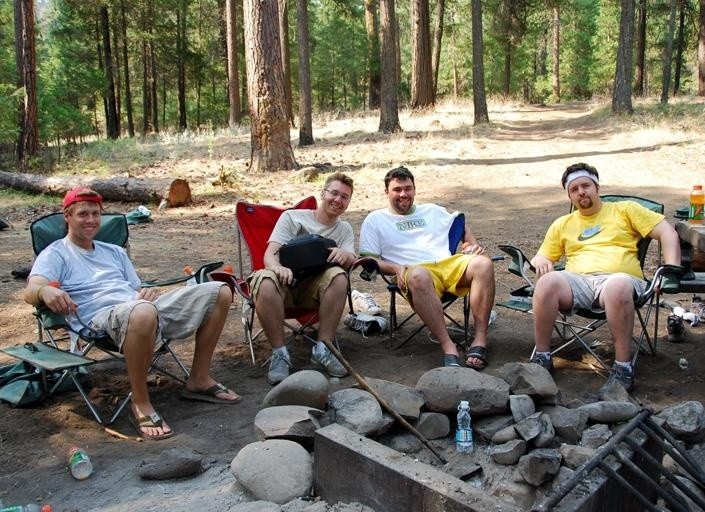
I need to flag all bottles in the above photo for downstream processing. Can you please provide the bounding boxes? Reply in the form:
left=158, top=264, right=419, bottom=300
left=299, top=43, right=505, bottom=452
left=691, top=297, right=705, bottom=323
left=667, top=316, right=685, bottom=342
left=50, top=281, right=60, bottom=288
left=463, top=240, right=474, bottom=254
left=456, top=400, right=475, bottom=453
left=224, top=261, right=233, bottom=273
left=0, top=502, right=51, bottom=512
left=184, top=266, right=198, bottom=285
left=68, top=445, right=93, bottom=479
left=689, top=184, right=705, bottom=225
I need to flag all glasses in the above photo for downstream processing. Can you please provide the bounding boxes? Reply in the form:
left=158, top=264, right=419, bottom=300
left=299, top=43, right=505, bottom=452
left=324, top=189, right=351, bottom=202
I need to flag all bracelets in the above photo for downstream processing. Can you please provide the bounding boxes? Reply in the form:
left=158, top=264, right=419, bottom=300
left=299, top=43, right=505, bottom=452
left=37, top=286, right=42, bottom=303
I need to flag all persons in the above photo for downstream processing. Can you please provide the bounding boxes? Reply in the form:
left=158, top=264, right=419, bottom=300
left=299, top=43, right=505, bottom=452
left=529, top=162, right=682, bottom=400
left=245, top=173, right=357, bottom=385
left=357, top=165, right=496, bottom=372
left=23, top=186, right=242, bottom=441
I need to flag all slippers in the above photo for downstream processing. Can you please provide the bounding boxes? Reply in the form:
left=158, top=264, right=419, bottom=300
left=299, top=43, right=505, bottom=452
left=180, top=382, right=242, bottom=404
left=442, top=353, right=462, bottom=369
left=465, top=345, right=489, bottom=370
left=128, top=408, right=175, bottom=440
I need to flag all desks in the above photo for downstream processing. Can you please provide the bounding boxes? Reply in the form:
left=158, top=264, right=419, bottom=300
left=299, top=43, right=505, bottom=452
left=675, top=220, right=705, bottom=251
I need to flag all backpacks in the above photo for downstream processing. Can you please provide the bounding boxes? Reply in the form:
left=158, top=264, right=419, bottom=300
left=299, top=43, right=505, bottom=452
left=276, top=232, right=343, bottom=274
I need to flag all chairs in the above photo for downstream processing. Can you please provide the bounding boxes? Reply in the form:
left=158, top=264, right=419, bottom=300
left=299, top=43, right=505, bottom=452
left=388, top=211, right=470, bottom=352
left=496, top=196, right=662, bottom=390
left=0, top=212, right=223, bottom=426
left=211, top=198, right=377, bottom=379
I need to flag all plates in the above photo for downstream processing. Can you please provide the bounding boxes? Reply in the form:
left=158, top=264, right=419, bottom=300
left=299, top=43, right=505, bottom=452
left=676, top=208, right=688, bottom=212
left=674, top=214, right=687, bottom=218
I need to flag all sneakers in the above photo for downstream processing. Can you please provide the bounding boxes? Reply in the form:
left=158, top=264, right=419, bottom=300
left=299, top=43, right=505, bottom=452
left=528, top=352, right=556, bottom=374
left=665, top=313, right=689, bottom=343
left=308, top=342, right=351, bottom=379
left=266, top=350, right=293, bottom=386
left=342, top=312, right=389, bottom=334
left=348, top=289, right=382, bottom=315
left=599, top=360, right=639, bottom=402
